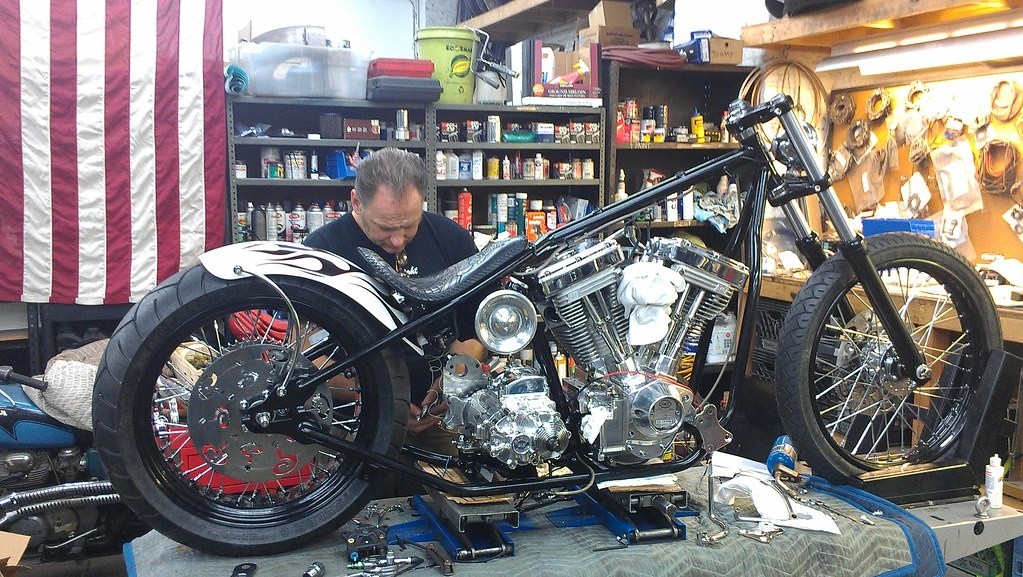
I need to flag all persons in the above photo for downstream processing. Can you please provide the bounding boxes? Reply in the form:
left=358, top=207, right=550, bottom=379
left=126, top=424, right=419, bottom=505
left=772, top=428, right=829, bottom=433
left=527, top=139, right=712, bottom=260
left=297, top=146, right=499, bottom=497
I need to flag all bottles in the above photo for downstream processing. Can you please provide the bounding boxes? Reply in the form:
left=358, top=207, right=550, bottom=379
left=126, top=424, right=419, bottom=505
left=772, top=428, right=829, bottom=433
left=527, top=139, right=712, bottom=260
left=520, top=340, right=575, bottom=387
left=437, top=149, right=594, bottom=247
left=616, top=96, right=739, bottom=144
left=717, top=171, right=740, bottom=219
left=235, top=194, right=349, bottom=244
left=984, top=453, right=1005, bottom=509
left=231, top=146, right=319, bottom=180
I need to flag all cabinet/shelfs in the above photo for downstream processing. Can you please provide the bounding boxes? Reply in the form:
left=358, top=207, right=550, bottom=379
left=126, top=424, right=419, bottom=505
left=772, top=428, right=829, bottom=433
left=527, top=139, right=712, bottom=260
left=607, top=56, right=763, bottom=262
left=223, top=91, right=607, bottom=272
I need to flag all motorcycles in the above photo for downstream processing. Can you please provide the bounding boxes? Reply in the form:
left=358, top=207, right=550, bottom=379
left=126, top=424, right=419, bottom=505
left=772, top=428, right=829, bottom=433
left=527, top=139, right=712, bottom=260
left=88, top=93, right=1002, bottom=559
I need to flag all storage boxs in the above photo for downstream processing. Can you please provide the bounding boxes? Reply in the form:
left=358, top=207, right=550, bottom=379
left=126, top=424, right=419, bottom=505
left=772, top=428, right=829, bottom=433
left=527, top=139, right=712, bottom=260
left=169, top=341, right=220, bottom=387
left=232, top=42, right=362, bottom=100
left=366, top=57, right=435, bottom=77
left=708, top=38, right=744, bottom=65
left=365, top=76, right=443, bottom=102
left=659, top=186, right=694, bottom=222
left=532, top=37, right=600, bottom=97
left=587, top=0, right=633, bottom=27
left=578, top=25, right=641, bottom=54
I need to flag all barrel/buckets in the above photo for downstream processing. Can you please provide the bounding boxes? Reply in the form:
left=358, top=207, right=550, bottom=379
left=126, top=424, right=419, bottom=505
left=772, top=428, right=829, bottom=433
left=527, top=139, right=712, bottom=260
left=414, top=26, right=481, bottom=105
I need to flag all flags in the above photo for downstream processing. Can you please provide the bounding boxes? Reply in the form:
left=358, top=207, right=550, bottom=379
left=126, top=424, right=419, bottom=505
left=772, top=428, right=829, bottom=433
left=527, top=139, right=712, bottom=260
left=0, top=0, right=227, bottom=306
